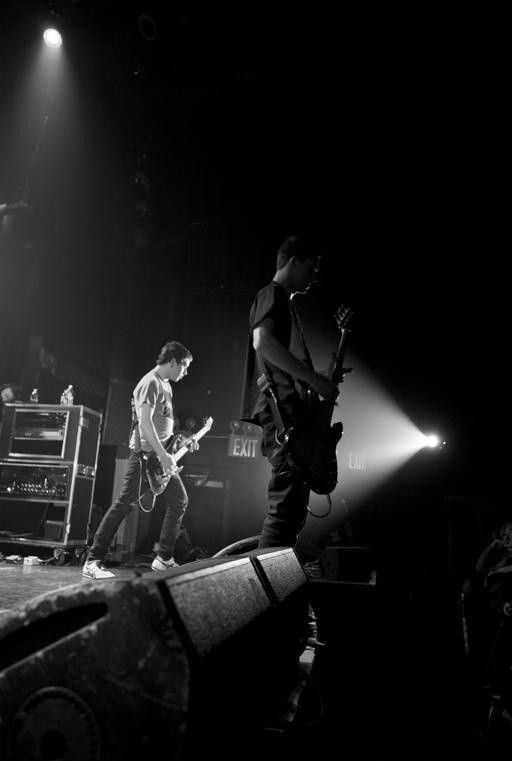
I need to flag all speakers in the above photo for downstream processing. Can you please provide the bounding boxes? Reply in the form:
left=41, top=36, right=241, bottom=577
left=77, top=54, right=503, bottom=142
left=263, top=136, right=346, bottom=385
left=0, top=573, right=192, bottom=761
left=237, top=543, right=308, bottom=603
left=143, top=554, right=277, bottom=656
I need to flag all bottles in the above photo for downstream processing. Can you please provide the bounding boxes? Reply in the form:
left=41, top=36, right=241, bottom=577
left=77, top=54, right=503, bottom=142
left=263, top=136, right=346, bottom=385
left=58, top=384, right=74, bottom=405
left=30, top=388, right=40, bottom=402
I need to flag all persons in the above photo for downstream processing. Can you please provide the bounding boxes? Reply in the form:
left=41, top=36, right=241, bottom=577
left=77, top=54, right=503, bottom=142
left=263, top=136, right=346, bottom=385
left=79, top=341, right=201, bottom=584
left=247, top=231, right=354, bottom=554
left=456, top=517, right=512, bottom=602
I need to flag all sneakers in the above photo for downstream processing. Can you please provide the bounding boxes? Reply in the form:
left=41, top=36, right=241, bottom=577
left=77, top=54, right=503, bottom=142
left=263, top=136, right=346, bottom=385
left=80, top=557, right=120, bottom=583
left=151, top=553, right=181, bottom=573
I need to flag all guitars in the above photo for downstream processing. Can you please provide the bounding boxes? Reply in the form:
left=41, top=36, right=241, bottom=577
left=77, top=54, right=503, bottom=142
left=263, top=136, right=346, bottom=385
left=285, top=304, right=353, bottom=494
left=142, top=416, right=214, bottom=496
